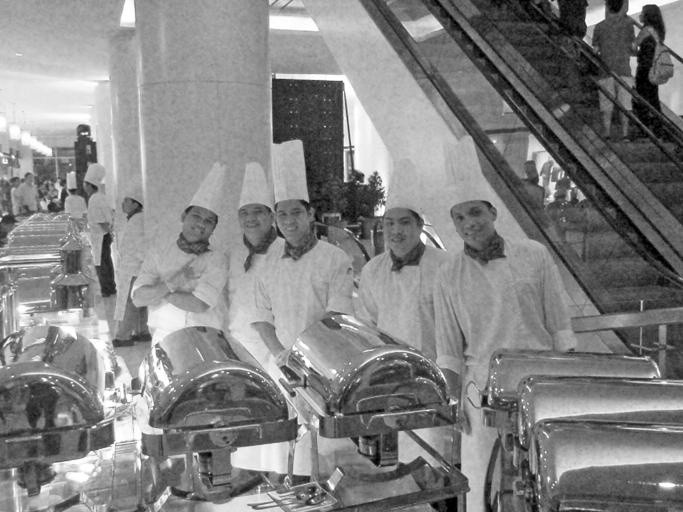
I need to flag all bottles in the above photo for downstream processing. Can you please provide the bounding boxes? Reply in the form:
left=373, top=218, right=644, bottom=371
left=108, top=438, right=143, bottom=512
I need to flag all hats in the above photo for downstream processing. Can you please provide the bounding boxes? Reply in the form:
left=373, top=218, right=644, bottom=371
left=126, top=180, right=143, bottom=206
left=84, top=163, right=105, bottom=187
left=446, top=135, right=495, bottom=213
left=67, top=171, right=77, bottom=190
left=189, top=140, right=309, bottom=216
left=384, top=159, right=420, bottom=218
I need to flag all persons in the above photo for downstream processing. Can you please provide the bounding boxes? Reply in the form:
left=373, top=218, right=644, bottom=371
left=224, top=205, right=286, bottom=496
left=431, top=200, right=580, bottom=512
left=130, top=204, right=228, bottom=331
left=0, top=172, right=117, bottom=298
left=111, top=197, right=152, bottom=347
left=250, top=199, right=354, bottom=484
left=556, top=1, right=667, bottom=140
left=355, top=205, right=457, bottom=512
left=520, top=175, right=590, bottom=266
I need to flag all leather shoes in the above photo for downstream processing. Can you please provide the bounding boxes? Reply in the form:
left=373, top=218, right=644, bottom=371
left=112, top=334, right=151, bottom=346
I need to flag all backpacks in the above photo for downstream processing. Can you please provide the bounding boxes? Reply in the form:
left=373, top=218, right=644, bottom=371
left=647, top=27, right=673, bottom=85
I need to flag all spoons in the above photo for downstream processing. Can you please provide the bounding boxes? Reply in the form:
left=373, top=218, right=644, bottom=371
left=247, top=485, right=327, bottom=511
